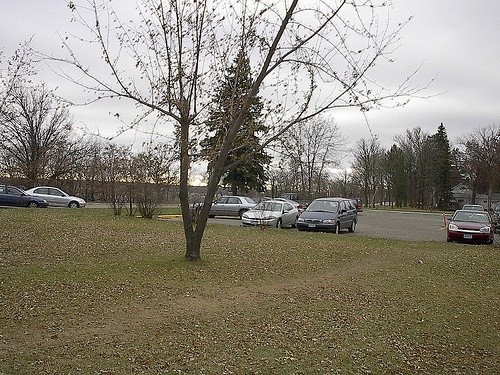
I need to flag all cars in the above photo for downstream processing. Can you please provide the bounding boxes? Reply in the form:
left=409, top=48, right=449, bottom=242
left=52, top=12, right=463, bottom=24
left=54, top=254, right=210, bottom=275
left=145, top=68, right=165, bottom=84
left=0, top=185, right=49, bottom=209
left=194, top=196, right=257, bottom=218
left=297, top=197, right=358, bottom=234
left=241, top=201, right=300, bottom=229
left=262, top=197, right=364, bottom=212
left=446, top=210, right=498, bottom=245
left=23, top=186, right=86, bottom=209
left=462, top=205, right=484, bottom=210
